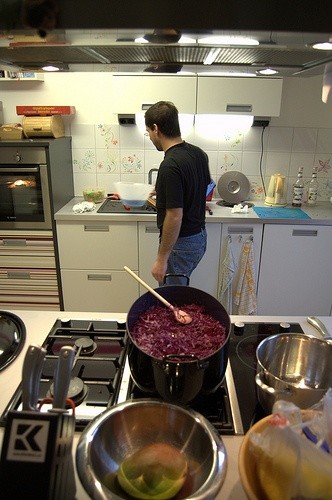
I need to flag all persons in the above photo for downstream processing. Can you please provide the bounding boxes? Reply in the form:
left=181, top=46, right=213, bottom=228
left=145, top=101, right=211, bottom=287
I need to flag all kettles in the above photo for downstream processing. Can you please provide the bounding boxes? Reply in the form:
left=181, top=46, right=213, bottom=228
left=263, top=173, right=288, bottom=208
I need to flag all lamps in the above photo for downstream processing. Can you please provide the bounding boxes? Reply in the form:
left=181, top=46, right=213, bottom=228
left=118, top=114, right=271, bottom=140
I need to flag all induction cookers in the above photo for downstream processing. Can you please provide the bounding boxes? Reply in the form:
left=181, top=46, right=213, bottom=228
left=227, top=322, right=308, bottom=435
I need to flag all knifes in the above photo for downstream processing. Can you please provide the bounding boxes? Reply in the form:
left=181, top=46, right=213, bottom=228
left=21, top=343, right=75, bottom=415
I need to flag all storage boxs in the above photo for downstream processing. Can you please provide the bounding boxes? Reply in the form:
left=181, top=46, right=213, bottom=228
left=15, top=105, right=76, bottom=116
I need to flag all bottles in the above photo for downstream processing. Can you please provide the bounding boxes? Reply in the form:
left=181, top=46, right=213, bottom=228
left=306, top=166, right=319, bottom=207
left=291, top=166, right=305, bottom=208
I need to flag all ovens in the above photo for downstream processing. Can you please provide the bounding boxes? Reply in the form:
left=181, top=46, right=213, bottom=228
left=0, top=135, right=74, bottom=231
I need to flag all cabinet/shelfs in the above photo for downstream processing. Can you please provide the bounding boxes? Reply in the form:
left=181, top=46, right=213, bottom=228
left=51, top=219, right=332, bottom=317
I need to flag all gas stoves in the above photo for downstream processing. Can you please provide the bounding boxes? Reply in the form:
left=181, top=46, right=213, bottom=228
left=0, top=317, right=236, bottom=435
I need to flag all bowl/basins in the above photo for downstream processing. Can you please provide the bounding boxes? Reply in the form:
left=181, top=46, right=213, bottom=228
left=76, top=397, right=228, bottom=500
left=83, top=185, right=104, bottom=203
left=114, top=181, right=155, bottom=208
left=237, top=408, right=332, bottom=500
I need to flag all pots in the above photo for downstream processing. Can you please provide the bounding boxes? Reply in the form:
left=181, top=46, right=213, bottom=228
left=254, top=332, right=332, bottom=412
left=126, top=272, right=232, bottom=405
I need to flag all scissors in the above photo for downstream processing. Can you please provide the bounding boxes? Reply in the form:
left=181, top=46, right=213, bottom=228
left=38, top=397, right=75, bottom=415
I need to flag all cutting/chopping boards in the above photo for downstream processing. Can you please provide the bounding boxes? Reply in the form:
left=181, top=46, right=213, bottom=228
left=253, top=207, right=311, bottom=219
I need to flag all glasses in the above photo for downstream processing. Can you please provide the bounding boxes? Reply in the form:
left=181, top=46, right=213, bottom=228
left=143, top=130, right=154, bottom=137
